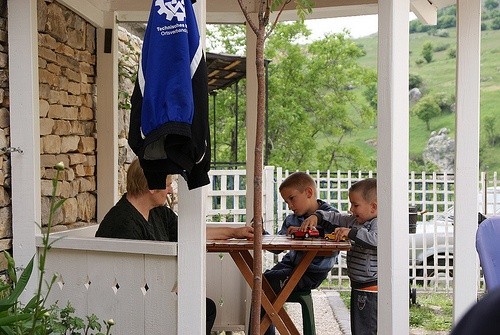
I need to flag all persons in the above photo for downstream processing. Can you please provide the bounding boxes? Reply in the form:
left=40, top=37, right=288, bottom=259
left=94, top=159, right=254, bottom=335
left=248, top=172, right=340, bottom=335
left=299, top=177, right=378, bottom=335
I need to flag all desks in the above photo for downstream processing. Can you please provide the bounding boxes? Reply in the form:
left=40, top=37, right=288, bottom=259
left=206, top=234, right=352, bottom=335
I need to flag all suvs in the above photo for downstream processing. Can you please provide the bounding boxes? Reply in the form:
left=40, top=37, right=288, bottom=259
left=324, top=232, right=345, bottom=242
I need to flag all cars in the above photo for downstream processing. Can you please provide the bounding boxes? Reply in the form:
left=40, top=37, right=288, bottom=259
left=291, top=226, right=319, bottom=240
